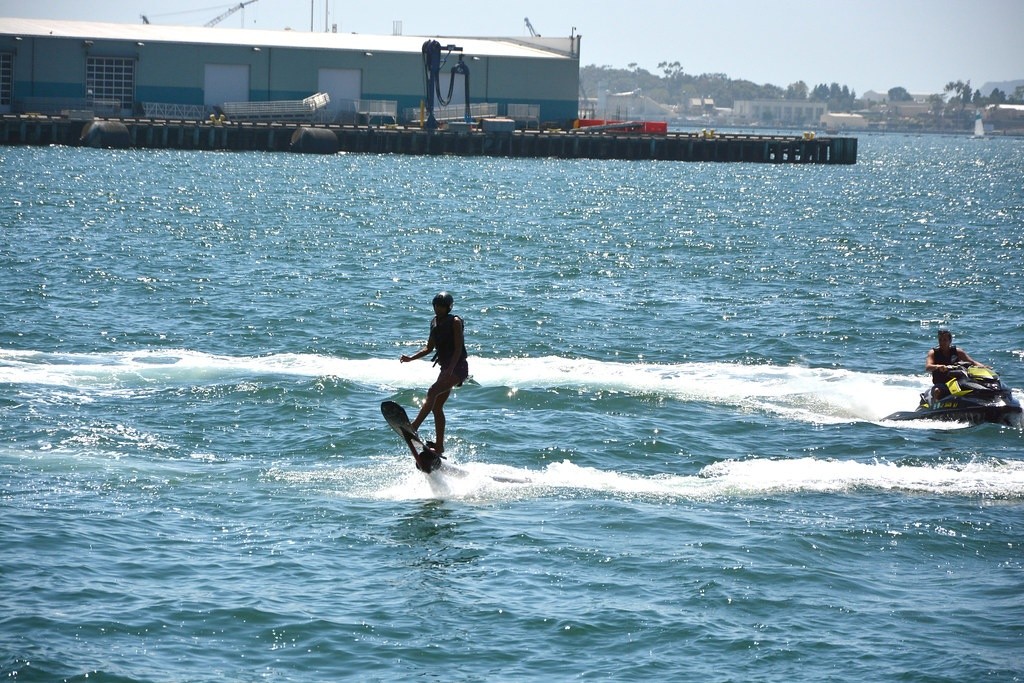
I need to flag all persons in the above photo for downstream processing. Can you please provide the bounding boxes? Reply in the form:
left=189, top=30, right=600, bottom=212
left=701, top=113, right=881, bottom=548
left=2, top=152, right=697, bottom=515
left=924, top=330, right=992, bottom=400
left=399, top=290, right=470, bottom=454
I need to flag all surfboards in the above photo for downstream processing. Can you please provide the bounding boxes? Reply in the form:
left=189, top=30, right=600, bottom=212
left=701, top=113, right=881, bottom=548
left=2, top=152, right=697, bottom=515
left=380, top=400, right=447, bottom=475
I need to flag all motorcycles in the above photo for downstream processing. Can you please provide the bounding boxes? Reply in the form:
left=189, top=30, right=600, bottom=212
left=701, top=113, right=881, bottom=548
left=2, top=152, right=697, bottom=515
left=878, top=362, right=1022, bottom=424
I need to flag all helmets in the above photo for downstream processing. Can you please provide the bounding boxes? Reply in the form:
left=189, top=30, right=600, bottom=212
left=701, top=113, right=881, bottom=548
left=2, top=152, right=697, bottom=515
left=432, top=291, right=454, bottom=313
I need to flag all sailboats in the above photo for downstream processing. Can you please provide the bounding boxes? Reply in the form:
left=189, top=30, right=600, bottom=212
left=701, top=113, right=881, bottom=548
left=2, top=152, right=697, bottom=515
left=970, top=109, right=989, bottom=141
